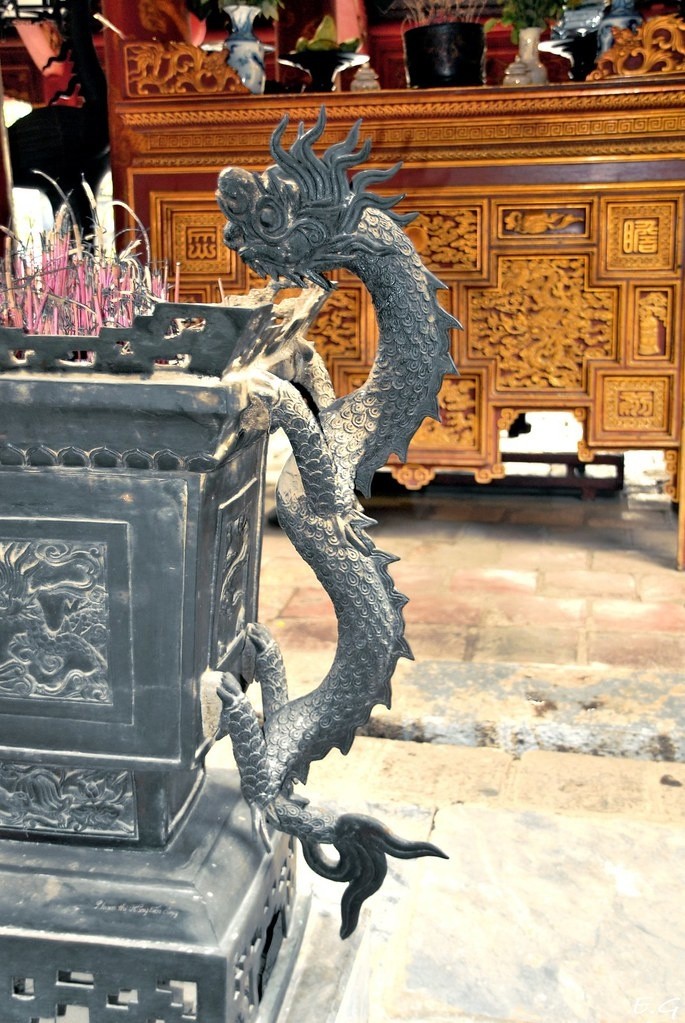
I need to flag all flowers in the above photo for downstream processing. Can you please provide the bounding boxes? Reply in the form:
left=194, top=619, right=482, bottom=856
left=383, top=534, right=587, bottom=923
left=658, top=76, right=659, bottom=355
left=385, top=0, right=487, bottom=26
left=199, top=0, right=285, bottom=20
left=483, top=0, right=570, bottom=45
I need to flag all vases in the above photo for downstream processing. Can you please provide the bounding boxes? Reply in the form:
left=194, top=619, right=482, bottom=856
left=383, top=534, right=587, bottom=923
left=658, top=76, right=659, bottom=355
left=220, top=5, right=267, bottom=95
left=403, top=21, right=486, bottom=88
left=502, top=27, right=556, bottom=84
